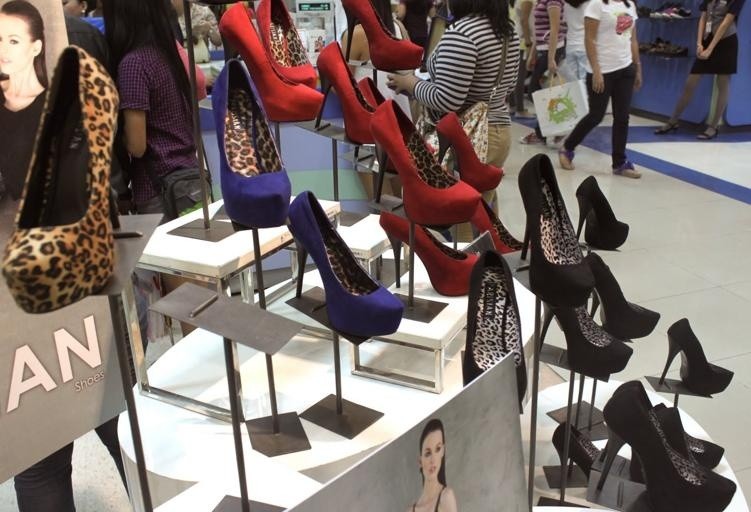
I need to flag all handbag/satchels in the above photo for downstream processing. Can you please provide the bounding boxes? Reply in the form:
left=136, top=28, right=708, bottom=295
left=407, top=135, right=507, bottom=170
left=415, top=93, right=492, bottom=170
left=530, top=73, right=591, bottom=138
left=155, top=163, right=215, bottom=221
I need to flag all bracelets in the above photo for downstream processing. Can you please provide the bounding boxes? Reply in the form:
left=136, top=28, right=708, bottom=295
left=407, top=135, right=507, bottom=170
left=696, top=44, right=702, bottom=48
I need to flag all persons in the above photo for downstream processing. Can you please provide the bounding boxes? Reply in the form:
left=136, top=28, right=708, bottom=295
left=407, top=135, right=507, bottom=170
left=13, top=288, right=146, bottom=511
left=654, top=0, right=744, bottom=139
left=59, top=1, right=643, bottom=227
left=0, top=0, right=48, bottom=200
left=411, top=418, right=457, bottom=512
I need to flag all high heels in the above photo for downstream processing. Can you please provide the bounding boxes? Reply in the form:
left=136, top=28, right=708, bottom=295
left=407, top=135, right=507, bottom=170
left=551, top=421, right=599, bottom=490
left=313, top=1, right=424, bottom=177
left=594, top=379, right=737, bottom=510
left=654, top=122, right=681, bottom=136
left=216, top=0, right=325, bottom=125
left=696, top=125, right=720, bottom=141
left=209, top=60, right=292, bottom=232
left=461, top=249, right=528, bottom=404
left=518, top=153, right=662, bottom=378
left=658, top=315, right=735, bottom=398
left=285, top=188, right=405, bottom=345
left=4, top=44, right=124, bottom=316
left=368, top=98, right=524, bottom=297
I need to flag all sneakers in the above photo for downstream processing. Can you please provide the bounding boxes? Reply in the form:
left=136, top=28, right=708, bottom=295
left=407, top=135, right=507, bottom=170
left=640, top=3, right=694, bottom=22
left=639, top=39, right=690, bottom=59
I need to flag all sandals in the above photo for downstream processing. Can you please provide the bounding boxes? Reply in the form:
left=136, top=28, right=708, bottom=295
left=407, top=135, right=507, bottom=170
left=557, top=148, right=575, bottom=170
left=613, top=160, right=644, bottom=179
